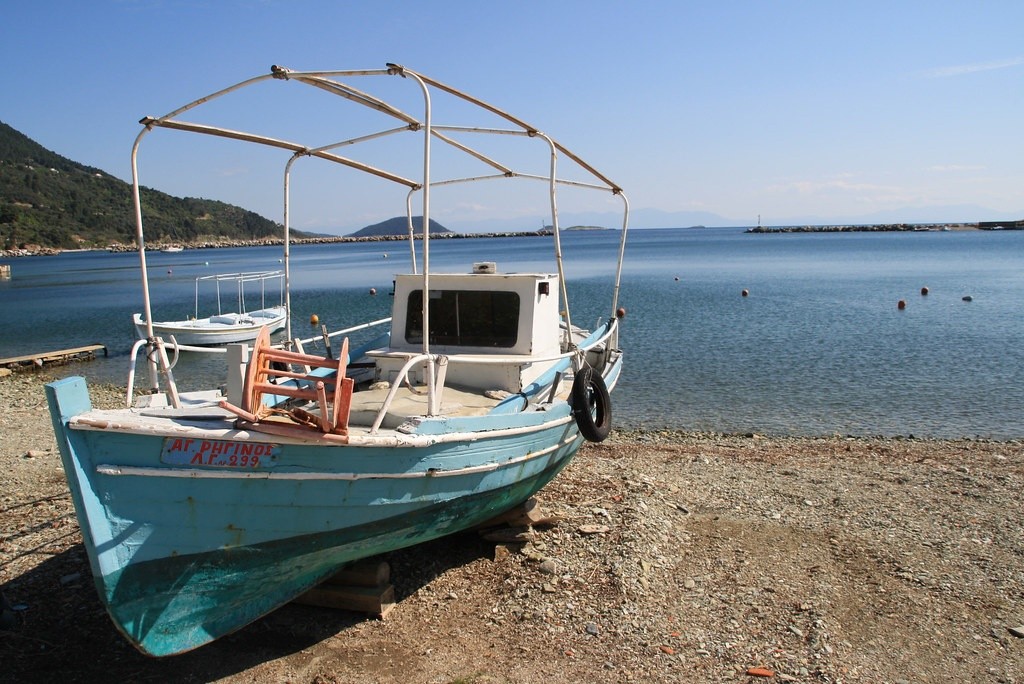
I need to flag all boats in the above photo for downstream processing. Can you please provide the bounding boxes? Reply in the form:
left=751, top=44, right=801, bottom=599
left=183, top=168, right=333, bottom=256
left=48, top=59, right=632, bottom=658
left=130, top=269, right=285, bottom=346
left=160, top=244, right=183, bottom=252
